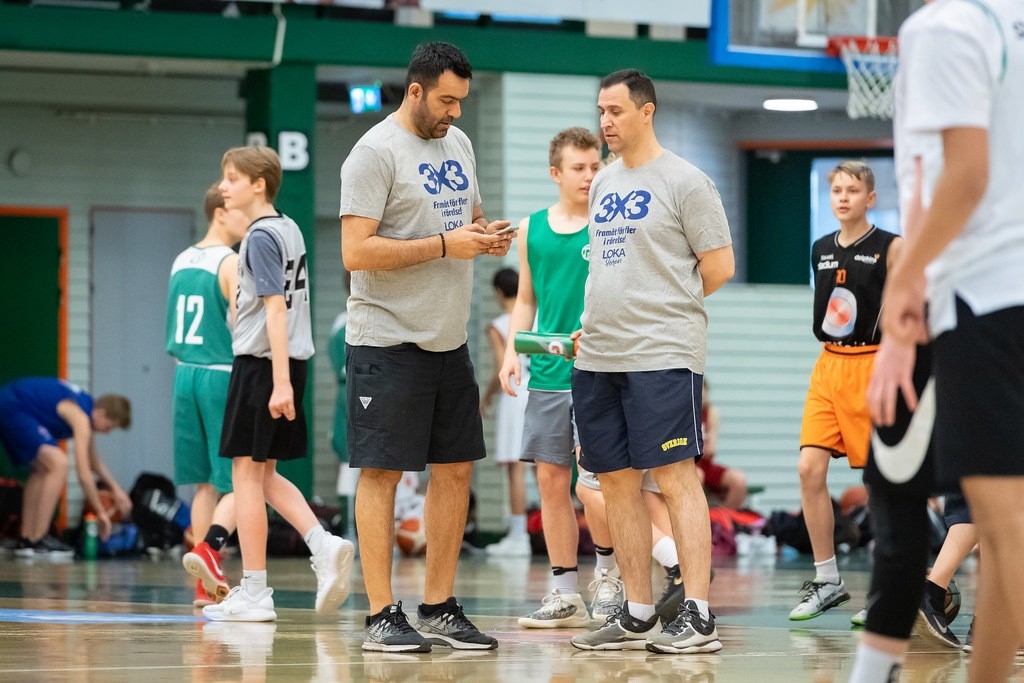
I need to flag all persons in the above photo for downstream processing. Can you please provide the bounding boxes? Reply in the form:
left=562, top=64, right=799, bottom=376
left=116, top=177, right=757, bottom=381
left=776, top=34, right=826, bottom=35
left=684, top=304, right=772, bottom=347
left=694, top=377, right=747, bottom=509
left=327, top=267, right=361, bottom=556
left=846, top=0, right=1024, bottom=683
left=498, top=67, right=735, bottom=654
left=0, top=377, right=133, bottom=556
left=340, top=41, right=518, bottom=652
left=201, top=145, right=355, bottom=621
left=164, top=179, right=251, bottom=608
left=481, top=266, right=539, bottom=555
left=789, top=160, right=904, bottom=625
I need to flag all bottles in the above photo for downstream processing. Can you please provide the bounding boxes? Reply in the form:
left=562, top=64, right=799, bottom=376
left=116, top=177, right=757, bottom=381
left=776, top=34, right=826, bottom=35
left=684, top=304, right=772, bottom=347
left=514, top=330, right=579, bottom=357
left=83, top=512, right=99, bottom=559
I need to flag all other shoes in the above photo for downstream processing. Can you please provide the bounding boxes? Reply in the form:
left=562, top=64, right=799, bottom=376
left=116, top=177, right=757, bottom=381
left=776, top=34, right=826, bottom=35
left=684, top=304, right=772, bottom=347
left=736, top=532, right=777, bottom=554
left=485, top=534, right=531, bottom=555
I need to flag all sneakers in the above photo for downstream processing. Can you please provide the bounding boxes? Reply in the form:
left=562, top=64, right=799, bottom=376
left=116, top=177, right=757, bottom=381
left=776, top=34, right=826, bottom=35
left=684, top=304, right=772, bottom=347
left=913, top=591, right=961, bottom=648
left=591, top=567, right=625, bottom=621
left=14, top=534, right=75, bottom=556
left=517, top=588, right=591, bottom=628
left=202, top=578, right=278, bottom=621
left=360, top=600, right=433, bottom=652
left=569, top=600, right=664, bottom=651
left=182, top=541, right=231, bottom=599
left=789, top=577, right=852, bottom=621
left=412, top=596, right=499, bottom=650
left=851, top=606, right=869, bottom=626
left=655, top=564, right=716, bottom=628
left=962, top=622, right=1024, bottom=656
left=309, top=531, right=355, bottom=614
left=645, top=599, right=723, bottom=654
left=192, top=577, right=223, bottom=607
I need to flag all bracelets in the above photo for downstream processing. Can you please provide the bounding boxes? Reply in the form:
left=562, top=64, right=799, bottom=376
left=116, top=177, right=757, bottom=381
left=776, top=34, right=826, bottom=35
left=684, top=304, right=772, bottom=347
left=439, top=232, right=446, bottom=258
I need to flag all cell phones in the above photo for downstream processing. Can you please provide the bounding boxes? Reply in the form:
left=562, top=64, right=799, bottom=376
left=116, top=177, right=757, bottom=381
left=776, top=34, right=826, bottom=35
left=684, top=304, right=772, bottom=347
left=493, top=226, right=519, bottom=236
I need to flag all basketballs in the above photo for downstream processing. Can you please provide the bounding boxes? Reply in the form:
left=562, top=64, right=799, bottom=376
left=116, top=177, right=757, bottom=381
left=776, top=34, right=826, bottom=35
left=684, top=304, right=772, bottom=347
left=926, top=566, right=960, bottom=626
left=397, top=519, right=427, bottom=557
left=839, top=488, right=868, bottom=516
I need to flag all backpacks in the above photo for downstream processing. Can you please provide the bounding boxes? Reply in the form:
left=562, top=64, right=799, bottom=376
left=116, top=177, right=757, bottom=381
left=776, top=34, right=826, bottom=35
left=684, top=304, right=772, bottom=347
left=709, top=506, right=737, bottom=555
left=130, top=473, right=192, bottom=547
